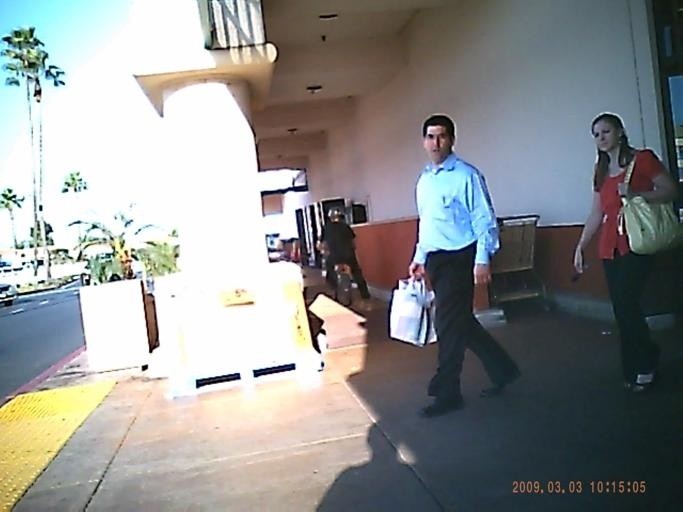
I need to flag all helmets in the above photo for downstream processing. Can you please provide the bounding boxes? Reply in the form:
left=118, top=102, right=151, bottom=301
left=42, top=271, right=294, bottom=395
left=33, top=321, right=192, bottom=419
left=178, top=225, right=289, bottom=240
left=327, top=207, right=343, bottom=218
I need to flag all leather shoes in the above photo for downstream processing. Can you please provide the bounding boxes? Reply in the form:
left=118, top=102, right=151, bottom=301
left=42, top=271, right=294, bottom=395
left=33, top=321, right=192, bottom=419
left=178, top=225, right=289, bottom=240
left=479, top=366, right=521, bottom=397
left=417, top=392, right=464, bottom=420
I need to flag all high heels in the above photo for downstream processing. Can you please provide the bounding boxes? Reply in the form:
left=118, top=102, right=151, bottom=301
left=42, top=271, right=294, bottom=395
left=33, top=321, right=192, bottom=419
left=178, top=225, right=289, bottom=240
left=621, top=365, right=659, bottom=392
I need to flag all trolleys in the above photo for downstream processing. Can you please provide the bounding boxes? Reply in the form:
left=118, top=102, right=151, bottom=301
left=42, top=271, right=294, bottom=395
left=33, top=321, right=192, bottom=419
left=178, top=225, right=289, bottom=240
left=489, top=212, right=555, bottom=322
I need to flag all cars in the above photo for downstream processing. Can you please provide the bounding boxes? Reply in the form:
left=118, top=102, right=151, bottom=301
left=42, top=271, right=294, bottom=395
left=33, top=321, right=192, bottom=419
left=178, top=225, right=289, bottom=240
left=0, top=251, right=74, bottom=278
left=0, top=283, right=17, bottom=309
left=81, top=256, right=147, bottom=285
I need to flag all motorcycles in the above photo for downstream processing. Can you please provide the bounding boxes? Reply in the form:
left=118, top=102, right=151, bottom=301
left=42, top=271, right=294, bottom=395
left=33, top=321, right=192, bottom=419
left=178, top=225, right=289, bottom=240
left=315, top=245, right=355, bottom=308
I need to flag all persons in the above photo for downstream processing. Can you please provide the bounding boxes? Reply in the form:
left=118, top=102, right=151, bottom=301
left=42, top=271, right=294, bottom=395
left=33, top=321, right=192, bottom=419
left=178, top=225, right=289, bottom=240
left=572, top=113, right=679, bottom=393
left=408, top=115, right=522, bottom=418
left=320, top=207, right=370, bottom=298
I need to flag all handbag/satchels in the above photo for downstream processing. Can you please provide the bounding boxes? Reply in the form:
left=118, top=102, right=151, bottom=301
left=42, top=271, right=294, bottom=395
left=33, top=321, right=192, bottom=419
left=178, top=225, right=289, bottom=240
left=617, top=154, right=682, bottom=254
left=388, top=276, right=438, bottom=347
